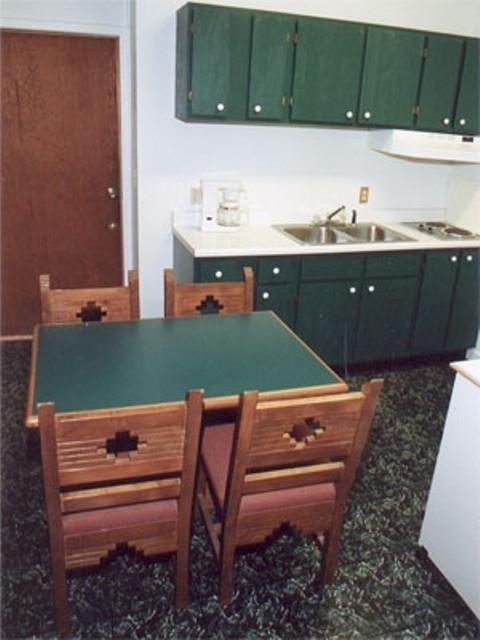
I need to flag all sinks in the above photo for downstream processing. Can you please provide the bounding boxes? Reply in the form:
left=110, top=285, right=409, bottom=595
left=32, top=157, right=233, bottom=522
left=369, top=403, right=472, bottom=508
left=270, top=224, right=358, bottom=247
left=334, top=220, right=418, bottom=244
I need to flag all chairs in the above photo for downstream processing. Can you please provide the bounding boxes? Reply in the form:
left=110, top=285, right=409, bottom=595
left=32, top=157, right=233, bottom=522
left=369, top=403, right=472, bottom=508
left=164, top=268, right=255, bottom=321
left=193, top=377, right=384, bottom=608
left=37, top=268, right=140, bottom=325
left=37, top=389, right=206, bottom=616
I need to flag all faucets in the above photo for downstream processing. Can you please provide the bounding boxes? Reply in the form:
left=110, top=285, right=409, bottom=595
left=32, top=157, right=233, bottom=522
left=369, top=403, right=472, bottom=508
left=320, top=203, right=347, bottom=224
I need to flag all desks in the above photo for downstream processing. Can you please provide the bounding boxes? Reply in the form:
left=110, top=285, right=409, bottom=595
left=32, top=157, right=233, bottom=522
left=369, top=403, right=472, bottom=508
left=20, top=310, right=350, bottom=431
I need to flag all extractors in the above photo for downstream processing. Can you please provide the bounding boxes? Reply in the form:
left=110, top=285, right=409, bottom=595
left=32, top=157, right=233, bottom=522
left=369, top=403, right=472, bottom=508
left=370, top=128, right=480, bottom=165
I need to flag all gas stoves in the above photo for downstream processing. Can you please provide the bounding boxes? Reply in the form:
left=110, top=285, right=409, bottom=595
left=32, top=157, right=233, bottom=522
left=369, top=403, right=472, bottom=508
left=399, top=221, right=480, bottom=240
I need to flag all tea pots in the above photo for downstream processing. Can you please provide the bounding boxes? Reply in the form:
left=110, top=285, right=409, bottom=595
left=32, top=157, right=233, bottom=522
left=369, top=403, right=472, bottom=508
left=215, top=185, right=248, bottom=228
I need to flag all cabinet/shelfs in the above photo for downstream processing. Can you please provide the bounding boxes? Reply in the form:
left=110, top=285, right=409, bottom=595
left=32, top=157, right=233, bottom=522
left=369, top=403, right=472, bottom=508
left=297, top=239, right=480, bottom=366
left=175, top=0, right=479, bottom=138
left=171, top=237, right=296, bottom=331
